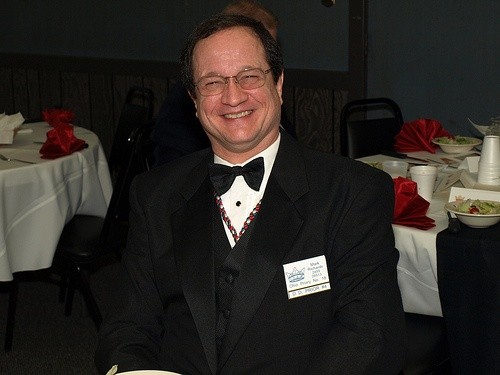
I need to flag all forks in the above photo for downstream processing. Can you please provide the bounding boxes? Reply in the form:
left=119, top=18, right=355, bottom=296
left=0, top=154, right=36, bottom=164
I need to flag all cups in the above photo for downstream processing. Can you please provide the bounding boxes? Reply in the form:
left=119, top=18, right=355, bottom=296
left=383, top=161, right=407, bottom=179
left=478, top=135, right=500, bottom=186
left=409, top=166, right=436, bottom=201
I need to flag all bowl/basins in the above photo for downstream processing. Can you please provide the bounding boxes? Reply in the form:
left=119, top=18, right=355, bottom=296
left=445, top=201, right=500, bottom=228
left=432, top=137, right=482, bottom=153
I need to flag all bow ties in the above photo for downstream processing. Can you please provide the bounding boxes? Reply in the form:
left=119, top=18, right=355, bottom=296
left=208, top=157, right=265, bottom=196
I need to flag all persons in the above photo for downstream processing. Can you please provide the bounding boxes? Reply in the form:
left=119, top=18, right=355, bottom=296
left=93, top=13, right=407, bottom=374
left=150, top=0, right=298, bottom=166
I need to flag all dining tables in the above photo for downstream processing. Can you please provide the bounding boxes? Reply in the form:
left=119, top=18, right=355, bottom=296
left=351, top=146, right=500, bottom=318
left=0, top=122, right=114, bottom=282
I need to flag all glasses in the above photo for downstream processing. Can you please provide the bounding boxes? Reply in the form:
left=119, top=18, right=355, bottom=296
left=191, top=68, right=272, bottom=96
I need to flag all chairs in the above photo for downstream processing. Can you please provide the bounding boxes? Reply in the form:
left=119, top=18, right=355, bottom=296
left=3, top=86, right=154, bottom=352
left=339, top=97, right=403, bottom=158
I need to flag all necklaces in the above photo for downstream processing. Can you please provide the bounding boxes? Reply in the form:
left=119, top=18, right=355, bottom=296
left=212, top=192, right=263, bottom=244
